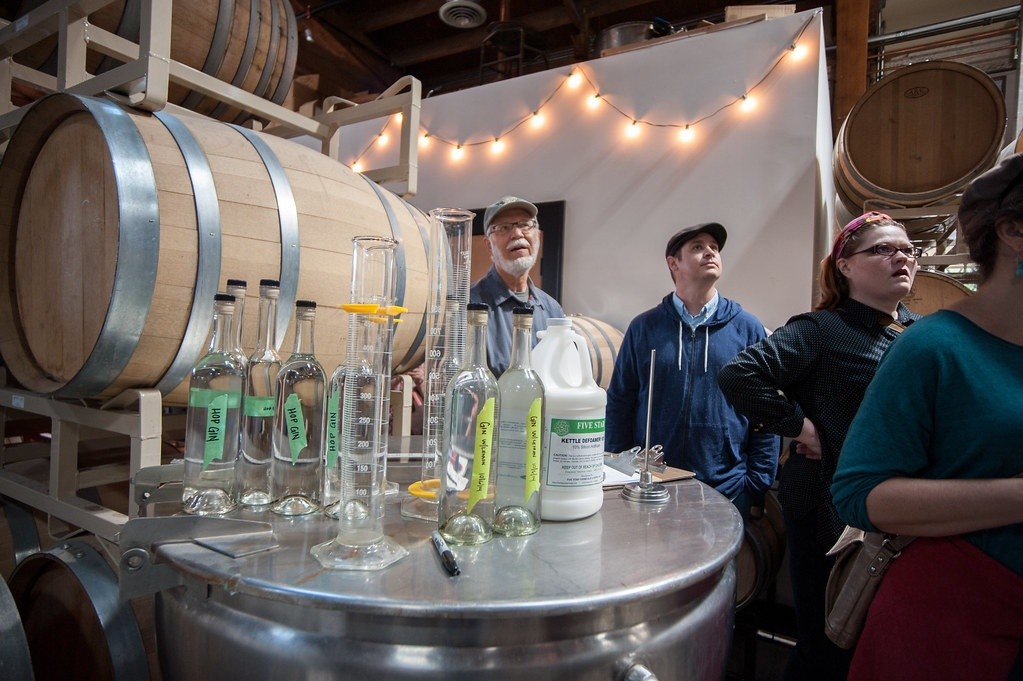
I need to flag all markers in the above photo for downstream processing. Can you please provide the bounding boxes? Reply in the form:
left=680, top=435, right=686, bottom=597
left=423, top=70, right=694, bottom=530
left=431, top=530, right=461, bottom=577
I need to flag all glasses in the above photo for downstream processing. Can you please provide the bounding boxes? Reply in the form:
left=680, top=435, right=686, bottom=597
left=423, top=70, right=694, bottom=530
left=487, top=219, right=538, bottom=233
left=843, top=241, right=922, bottom=260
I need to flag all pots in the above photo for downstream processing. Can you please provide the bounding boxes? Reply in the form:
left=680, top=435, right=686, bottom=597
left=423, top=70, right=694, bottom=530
left=591, top=18, right=676, bottom=60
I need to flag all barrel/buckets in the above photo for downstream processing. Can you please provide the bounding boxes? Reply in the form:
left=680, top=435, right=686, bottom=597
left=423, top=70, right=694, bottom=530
left=0, top=102, right=456, bottom=415
left=0, top=439, right=188, bottom=588
left=7, top=518, right=167, bottom=681
left=890, top=266, right=982, bottom=323
left=9, top=0, right=299, bottom=132
left=0, top=562, right=39, bottom=681
left=831, top=58, right=1011, bottom=238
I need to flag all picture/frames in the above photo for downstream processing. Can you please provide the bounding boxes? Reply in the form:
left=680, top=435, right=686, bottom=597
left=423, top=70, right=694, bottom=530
left=427, top=200, right=566, bottom=308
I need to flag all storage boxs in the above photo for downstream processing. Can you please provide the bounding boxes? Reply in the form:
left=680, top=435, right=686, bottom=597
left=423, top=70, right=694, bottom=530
left=724, top=4, right=797, bottom=22
left=265, top=74, right=379, bottom=128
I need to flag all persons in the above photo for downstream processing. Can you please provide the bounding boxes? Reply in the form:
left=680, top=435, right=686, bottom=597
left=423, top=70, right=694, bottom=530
left=831, top=154, right=1023, bottom=681
left=604, top=222, right=780, bottom=523
left=716, top=212, right=925, bottom=681
left=442, top=196, right=567, bottom=381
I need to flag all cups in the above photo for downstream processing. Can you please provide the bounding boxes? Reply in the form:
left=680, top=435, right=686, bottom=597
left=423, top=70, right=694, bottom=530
left=308, top=207, right=480, bottom=575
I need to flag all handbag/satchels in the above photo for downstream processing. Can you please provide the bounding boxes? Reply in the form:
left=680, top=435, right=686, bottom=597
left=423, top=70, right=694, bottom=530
left=734, top=489, right=787, bottom=611
left=824, top=525, right=901, bottom=650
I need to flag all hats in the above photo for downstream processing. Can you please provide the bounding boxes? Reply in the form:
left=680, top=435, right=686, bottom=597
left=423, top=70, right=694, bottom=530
left=665, top=222, right=727, bottom=258
left=483, top=196, right=538, bottom=236
left=957, top=155, right=1023, bottom=242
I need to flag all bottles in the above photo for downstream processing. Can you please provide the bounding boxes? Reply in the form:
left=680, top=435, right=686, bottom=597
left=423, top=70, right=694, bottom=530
left=239, top=280, right=286, bottom=506
left=205, top=279, right=249, bottom=410
left=437, top=302, right=497, bottom=546
left=522, top=316, right=606, bottom=521
left=492, top=305, right=544, bottom=537
left=321, top=303, right=384, bottom=520
left=267, top=299, right=330, bottom=516
left=181, top=294, right=244, bottom=517
left=441, top=286, right=466, bottom=436
